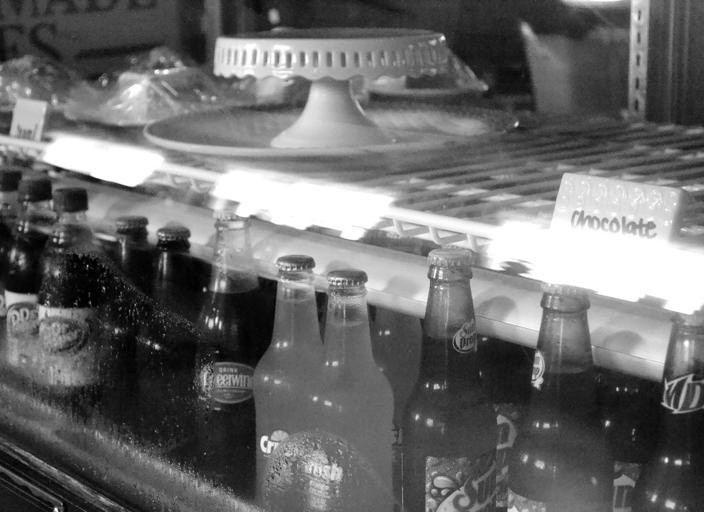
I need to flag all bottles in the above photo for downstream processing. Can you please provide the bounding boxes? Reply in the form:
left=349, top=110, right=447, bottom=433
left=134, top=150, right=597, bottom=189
left=0, top=163, right=270, bottom=497
left=249, top=244, right=704, bottom=511
